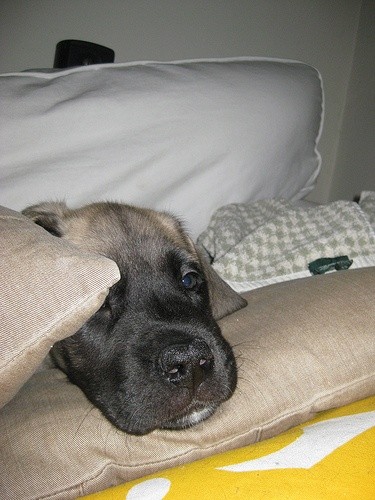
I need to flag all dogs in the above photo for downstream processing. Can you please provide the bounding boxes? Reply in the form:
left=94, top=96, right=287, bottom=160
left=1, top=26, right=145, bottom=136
left=19, top=200, right=249, bottom=460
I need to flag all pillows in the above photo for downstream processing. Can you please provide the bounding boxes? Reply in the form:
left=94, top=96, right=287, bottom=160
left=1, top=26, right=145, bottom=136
left=0, top=205, right=120, bottom=408
